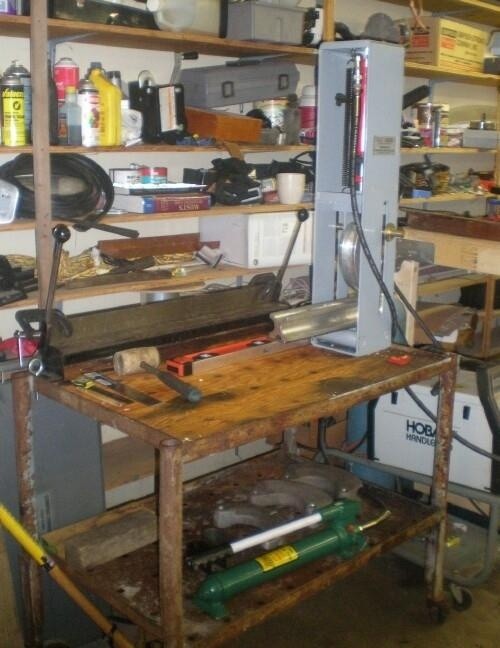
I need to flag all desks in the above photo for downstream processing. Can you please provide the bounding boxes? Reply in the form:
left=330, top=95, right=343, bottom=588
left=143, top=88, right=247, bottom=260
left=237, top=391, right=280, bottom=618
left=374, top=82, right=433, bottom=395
left=10, top=341, right=462, bottom=648
left=400, top=206, right=499, bottom=278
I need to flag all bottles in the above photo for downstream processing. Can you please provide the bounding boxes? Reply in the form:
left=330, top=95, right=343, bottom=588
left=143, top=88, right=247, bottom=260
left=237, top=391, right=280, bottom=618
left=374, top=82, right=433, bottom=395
left=416, top=101, right=451, bottom=148
left=0, top=59, right=123, bottom=148
left=298, top=83, right=317, bottom=145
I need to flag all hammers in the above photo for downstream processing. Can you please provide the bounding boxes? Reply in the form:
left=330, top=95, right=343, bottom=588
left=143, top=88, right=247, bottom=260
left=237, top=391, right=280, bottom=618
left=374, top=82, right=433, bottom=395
left=113, top=346, right=201, bottom=403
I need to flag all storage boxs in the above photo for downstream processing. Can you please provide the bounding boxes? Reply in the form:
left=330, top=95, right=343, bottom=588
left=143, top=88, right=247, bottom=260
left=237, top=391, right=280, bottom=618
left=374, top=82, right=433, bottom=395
left=404, top=16, right=491, bottom=71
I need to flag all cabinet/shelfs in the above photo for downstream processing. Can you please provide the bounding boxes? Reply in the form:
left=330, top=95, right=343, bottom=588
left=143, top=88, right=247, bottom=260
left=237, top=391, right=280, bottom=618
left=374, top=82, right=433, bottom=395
left=1, top=0, right=334, bottom=310
left=387, top=0, right=500, bottom=360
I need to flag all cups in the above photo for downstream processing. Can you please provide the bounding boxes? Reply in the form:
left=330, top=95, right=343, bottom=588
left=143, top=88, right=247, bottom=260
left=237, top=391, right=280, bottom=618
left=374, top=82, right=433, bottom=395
left=275, top=172, right=307, bottom=204
